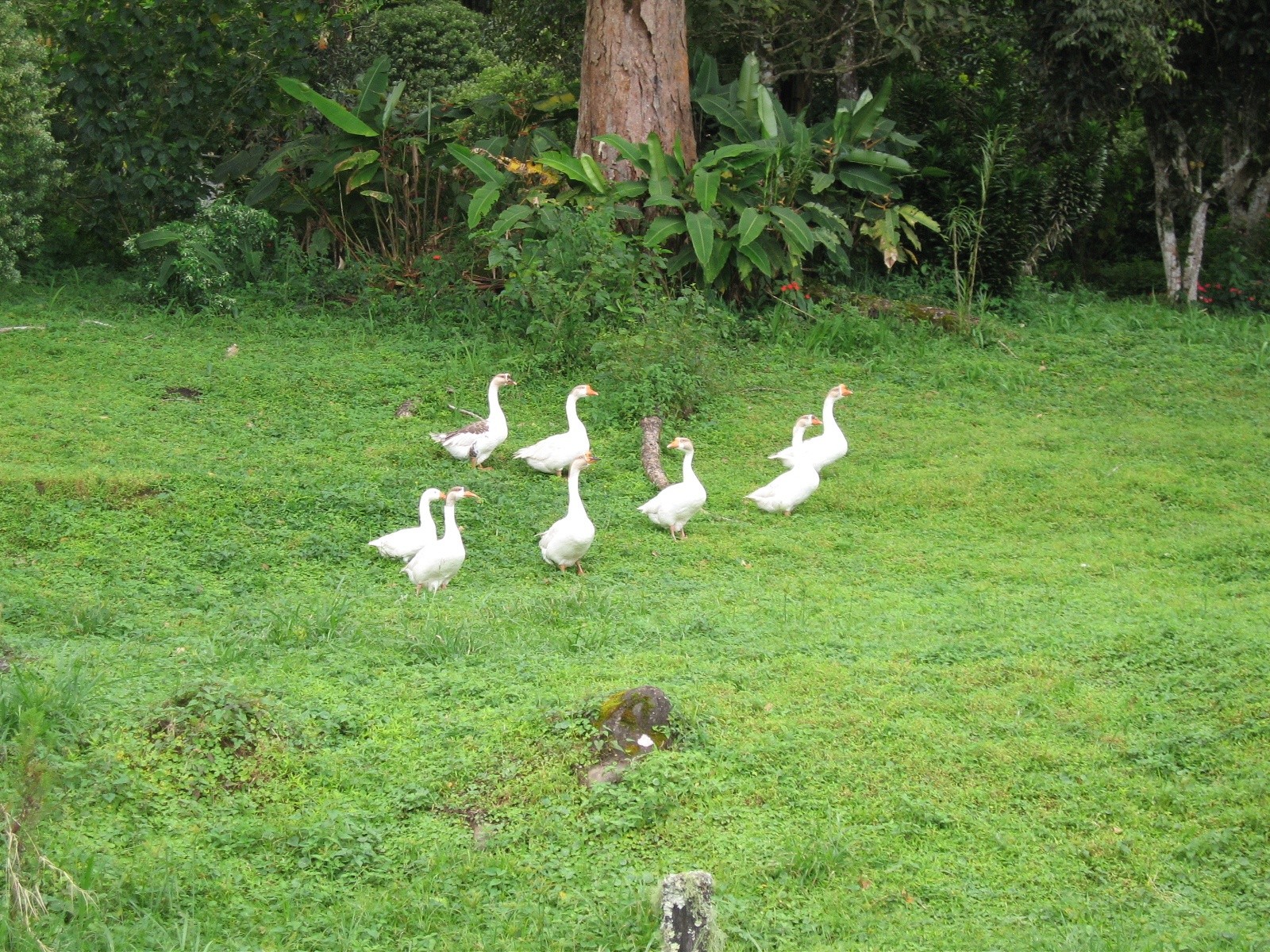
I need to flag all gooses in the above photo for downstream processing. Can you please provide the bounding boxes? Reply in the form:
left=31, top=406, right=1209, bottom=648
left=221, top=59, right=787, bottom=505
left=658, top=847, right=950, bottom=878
left=399, top=486, right=480, bottom=595
left=637, top=436, right=707, bottom=544
left=428, top=373, right=518, bottom=471
left=513, top=384, right=599, bottom=479
left=532, top=451, right=602, bottom=575
left=743, top=414, right=823, bottom=518
left=768, top=383, right=854, bottom=475
left=367, top=487, right=447, bottom=562
